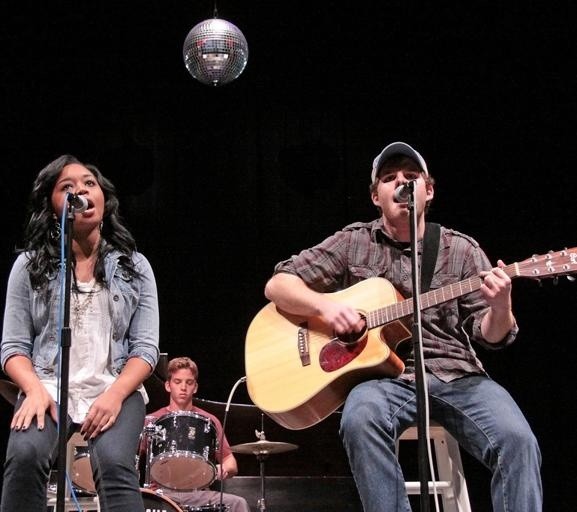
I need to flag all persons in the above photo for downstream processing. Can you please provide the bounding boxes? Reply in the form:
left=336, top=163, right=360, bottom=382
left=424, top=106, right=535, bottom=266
left=0, top=152, right=161, bottom=511
left=263, top=140, right=545, bottom=510
left=138, top=357, right=250, bottom=511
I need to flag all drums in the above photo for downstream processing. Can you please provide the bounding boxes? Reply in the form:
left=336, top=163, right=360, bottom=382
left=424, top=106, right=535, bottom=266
left=137, top=487, right=184, bottom=512
left=144, top=410, right=219, bottom=491
left=69, top=447, right=98, bottom=495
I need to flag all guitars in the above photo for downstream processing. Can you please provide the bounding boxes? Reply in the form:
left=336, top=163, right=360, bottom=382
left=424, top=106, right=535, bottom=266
left=245, top=247, right=577, bottom=432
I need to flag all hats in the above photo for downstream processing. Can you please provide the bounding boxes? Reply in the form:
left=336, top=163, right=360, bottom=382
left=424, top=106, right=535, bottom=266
left=371, top=141, right=430, bottom=183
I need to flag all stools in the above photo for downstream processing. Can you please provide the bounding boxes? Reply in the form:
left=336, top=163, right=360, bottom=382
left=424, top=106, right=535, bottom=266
left=396, top=422, right=472, bottom=511
left=49, top=433, right=101, bottom=512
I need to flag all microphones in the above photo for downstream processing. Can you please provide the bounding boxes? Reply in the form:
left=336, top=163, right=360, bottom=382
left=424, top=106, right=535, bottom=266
left=391, top=181, right=417, bottom=204
left=66, top=189, right=90, bottom=216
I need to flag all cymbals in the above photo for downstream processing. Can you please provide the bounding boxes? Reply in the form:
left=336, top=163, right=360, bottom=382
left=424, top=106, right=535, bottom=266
left=227, top=439, right=297, bottom=456
